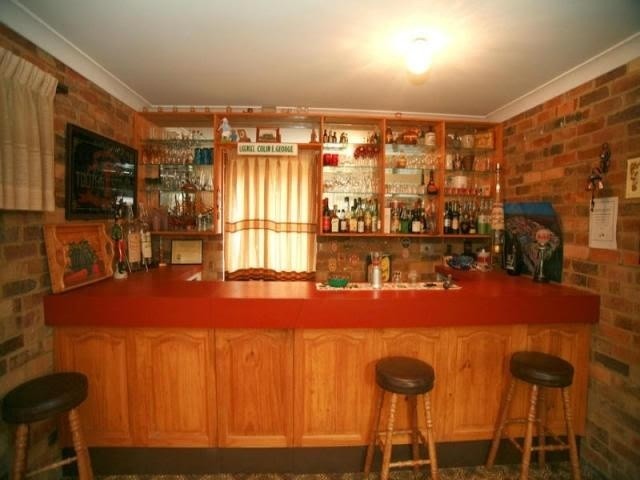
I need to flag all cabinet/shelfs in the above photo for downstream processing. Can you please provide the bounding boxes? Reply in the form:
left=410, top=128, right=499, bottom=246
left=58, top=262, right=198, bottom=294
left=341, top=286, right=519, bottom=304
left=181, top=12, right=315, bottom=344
left=319, top=116, right=503, bottom=238
left=132, top=109, right=223, bottom=238
left=224, top=110, right=323, bottom=148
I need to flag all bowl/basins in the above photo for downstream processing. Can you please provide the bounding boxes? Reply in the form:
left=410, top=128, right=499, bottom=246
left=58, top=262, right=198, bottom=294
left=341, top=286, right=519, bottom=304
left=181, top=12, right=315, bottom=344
left=328, top=278, right=349, bottom=287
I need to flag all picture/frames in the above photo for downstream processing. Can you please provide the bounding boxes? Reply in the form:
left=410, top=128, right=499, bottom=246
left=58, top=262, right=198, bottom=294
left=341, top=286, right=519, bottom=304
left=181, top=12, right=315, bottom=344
left=170, top=238, right=204, bottom=265
left=255, top=126, right=280, bottom=144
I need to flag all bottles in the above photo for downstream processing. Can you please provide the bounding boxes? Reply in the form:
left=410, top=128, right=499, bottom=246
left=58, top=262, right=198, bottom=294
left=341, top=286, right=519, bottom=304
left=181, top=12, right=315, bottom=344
left=534, top=243, right=550, bottom=282
left=374, top=132, right=378, bottom=143
left=427, top=170, right=437, bottom=194
left=340, top=133, right=348, bottom=143
left=329, top=131, right=332, bottom=143
left=138, top=199, right=153, bottom=267
left=384, top=200, right=435, bottom=234
left=111, top=210, right=125, bottom=271
left=124, top=201, right=141, bottom=271
left=444, top=200, right=492, bottom=234
left=505, top=232, right=522, bottom=276
left=454, top=154, right=462, bottom=171
left=391, top=271, right=402, bottom=289
left=324, top=129, right=328, bottom=143
left=372, top=252, right=382, bottom=289
left=386, top=127, right=393, bottom=144
left=333, top=132, right=337, bottom=143
left=323, top=197, right=381, bottom=233
left=195, top=148, right=213, bottom=165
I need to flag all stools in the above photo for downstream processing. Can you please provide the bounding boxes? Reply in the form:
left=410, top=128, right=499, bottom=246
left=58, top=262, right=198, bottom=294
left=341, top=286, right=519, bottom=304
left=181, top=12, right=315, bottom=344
left=486, top=350, right=580, bottom=480
left=0, top=371, right=95, bottom=479
left=364, top=355, right=439, bottom=480
